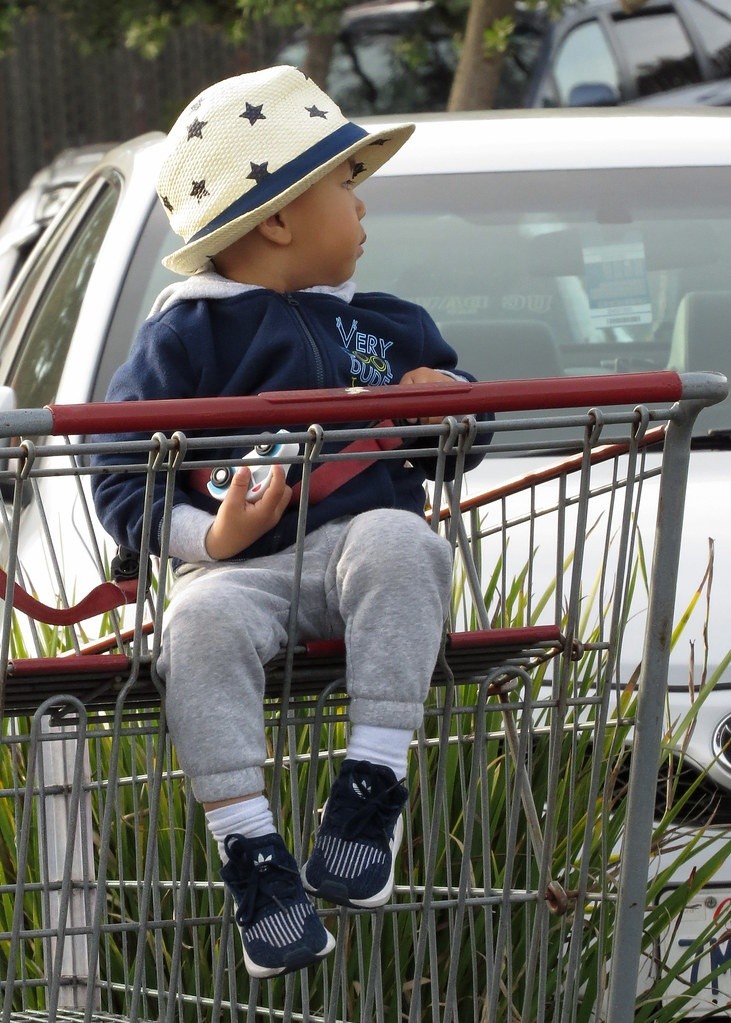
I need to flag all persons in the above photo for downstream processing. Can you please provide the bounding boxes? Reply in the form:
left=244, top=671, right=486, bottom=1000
left=89, top=65, right=497, bottom=980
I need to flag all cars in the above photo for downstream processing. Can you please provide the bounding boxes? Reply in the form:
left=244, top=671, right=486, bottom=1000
left=0, top=0, right=731, bottom=1021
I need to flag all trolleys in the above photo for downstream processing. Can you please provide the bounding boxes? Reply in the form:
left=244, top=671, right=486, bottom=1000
left=0, top=368, right=731, bottom=1021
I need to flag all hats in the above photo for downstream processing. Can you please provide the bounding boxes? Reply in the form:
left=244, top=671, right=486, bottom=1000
left=154, top=64, right=417, bottom=277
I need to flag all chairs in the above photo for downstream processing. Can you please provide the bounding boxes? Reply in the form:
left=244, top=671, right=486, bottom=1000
left=427, top=318, right=567, bottom=387
left=666, top=292, right=731, bottom=383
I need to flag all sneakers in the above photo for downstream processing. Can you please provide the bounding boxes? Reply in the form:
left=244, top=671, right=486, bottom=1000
left=218, top=833, right=335, bottom=979
left=300, top=759, right=409, bottom=909
